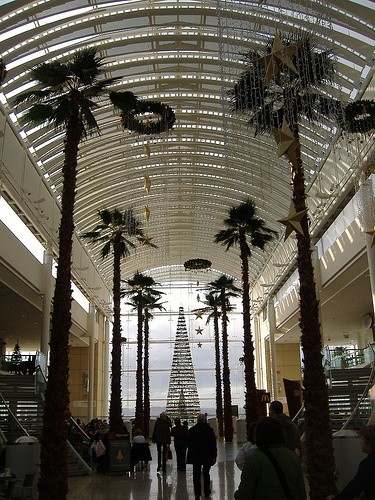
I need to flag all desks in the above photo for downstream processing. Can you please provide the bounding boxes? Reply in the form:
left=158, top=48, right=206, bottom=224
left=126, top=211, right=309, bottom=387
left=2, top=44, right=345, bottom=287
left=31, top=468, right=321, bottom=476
left=0, top=474, right=16, bottom=500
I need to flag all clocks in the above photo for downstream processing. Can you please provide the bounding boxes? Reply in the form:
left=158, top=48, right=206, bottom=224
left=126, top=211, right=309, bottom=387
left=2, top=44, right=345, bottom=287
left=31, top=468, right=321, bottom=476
left=361, top=314, right=373, bottom=328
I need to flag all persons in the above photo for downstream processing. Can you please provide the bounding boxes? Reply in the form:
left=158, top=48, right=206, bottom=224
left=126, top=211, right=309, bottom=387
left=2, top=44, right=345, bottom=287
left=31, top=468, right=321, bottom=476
left=269, top=401, right=303, bottom=458
left=74, top=412, right=188, bottom=472
left=234, top=417, right=307, bottom=500
left=184, top=414, right=217, bottom=500
left=326, top=426, right=375, bottom=500
left=235, top=422, right=258, bottom=472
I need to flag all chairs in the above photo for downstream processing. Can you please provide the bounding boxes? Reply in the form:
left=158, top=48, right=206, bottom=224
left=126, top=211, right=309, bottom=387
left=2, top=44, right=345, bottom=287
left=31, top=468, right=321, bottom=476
left=131, top=443, right=152, bottom=472
left=14, top=471, right=36, bottom=500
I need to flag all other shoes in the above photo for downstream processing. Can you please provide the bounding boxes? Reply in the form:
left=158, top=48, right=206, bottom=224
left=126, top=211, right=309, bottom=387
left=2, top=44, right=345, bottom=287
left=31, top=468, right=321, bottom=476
left=157, top=467, right=160, bottom=471
left=205, top=491, right=211, bottom=496
left=194, top=495, right=200, bottom=500
left=162, top=467, right=166, bottom=472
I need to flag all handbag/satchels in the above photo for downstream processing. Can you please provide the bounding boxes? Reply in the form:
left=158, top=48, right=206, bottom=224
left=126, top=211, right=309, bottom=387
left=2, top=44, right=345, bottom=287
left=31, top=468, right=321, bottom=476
left=165, top=443, right=172, bottom=460
